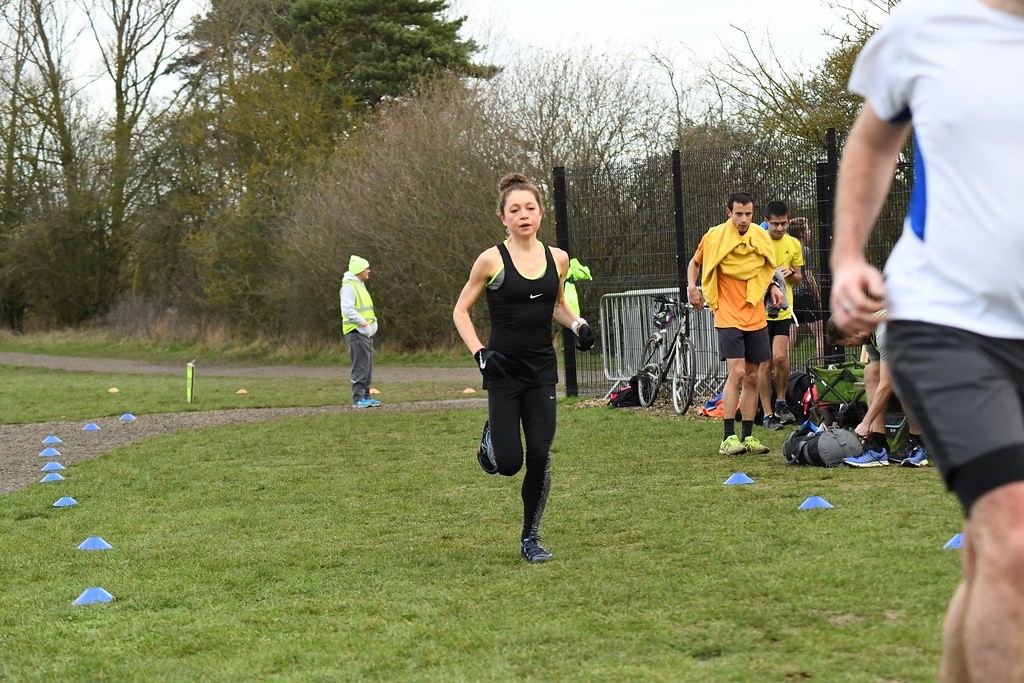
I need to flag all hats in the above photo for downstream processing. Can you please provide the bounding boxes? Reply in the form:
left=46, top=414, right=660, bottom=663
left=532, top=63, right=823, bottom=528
left=348, top=254, right=370, bottom=274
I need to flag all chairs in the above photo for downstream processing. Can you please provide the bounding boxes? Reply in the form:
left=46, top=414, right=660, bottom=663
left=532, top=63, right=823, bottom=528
left=805, top=352, right=865, bottom=427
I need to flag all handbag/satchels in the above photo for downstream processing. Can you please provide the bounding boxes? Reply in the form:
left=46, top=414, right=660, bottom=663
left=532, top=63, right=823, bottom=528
left=780, top=423, right=863, bottom=468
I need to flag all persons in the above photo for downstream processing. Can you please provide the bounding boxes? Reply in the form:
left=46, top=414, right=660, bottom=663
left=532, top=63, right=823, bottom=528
left=759, top=201, right=803, bottom=432
left=830, top=0, right=1024, bottom=683
left=339, top=254, right=382, bottom=408
left=789, top=217, right=825, bottom=369
left=452, top=175, right=595, bottom=564
left=830, top=311, right=929, bottom=469
left=687, top=192, right=777, bottom=458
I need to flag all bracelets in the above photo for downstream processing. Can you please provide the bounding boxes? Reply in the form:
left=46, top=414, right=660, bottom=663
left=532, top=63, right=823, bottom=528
left=572, top=318, right=589, bottom=336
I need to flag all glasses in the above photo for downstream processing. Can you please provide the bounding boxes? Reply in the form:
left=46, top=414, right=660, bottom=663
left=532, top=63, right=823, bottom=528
left=767, top=219, right=788, bottom=227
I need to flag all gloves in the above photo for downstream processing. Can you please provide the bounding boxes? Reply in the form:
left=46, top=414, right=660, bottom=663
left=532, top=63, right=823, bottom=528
left=577, top=324, right=595, bottom=351
left=474, top=348, right=506, bottom=381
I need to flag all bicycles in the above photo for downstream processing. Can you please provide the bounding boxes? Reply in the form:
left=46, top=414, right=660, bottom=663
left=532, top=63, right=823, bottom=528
left=634, top=294, right=712, bottom=415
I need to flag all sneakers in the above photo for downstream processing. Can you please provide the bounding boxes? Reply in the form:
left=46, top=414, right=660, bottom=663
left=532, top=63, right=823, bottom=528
left=842, top=447, right=890, bottom=468
left=477, top=418, right=496, bottom=475
left=899, top=441, right=935, bottom=467
left=887, top=437, right=920, bottom=462
left=774, top=401, right=796, bottom=423
left=742, top=435, right=770, bottom=455
left=366, top=399, right=382, bottom=406
left=860, top=432, right=889, bottom=456
left=352, top=399, right=372, bottom=408
left=763, top=415, right=783, bottom=430
left=718, top=434, right=747, bottom=455
left=521, top=534, right=552, bottom=562
